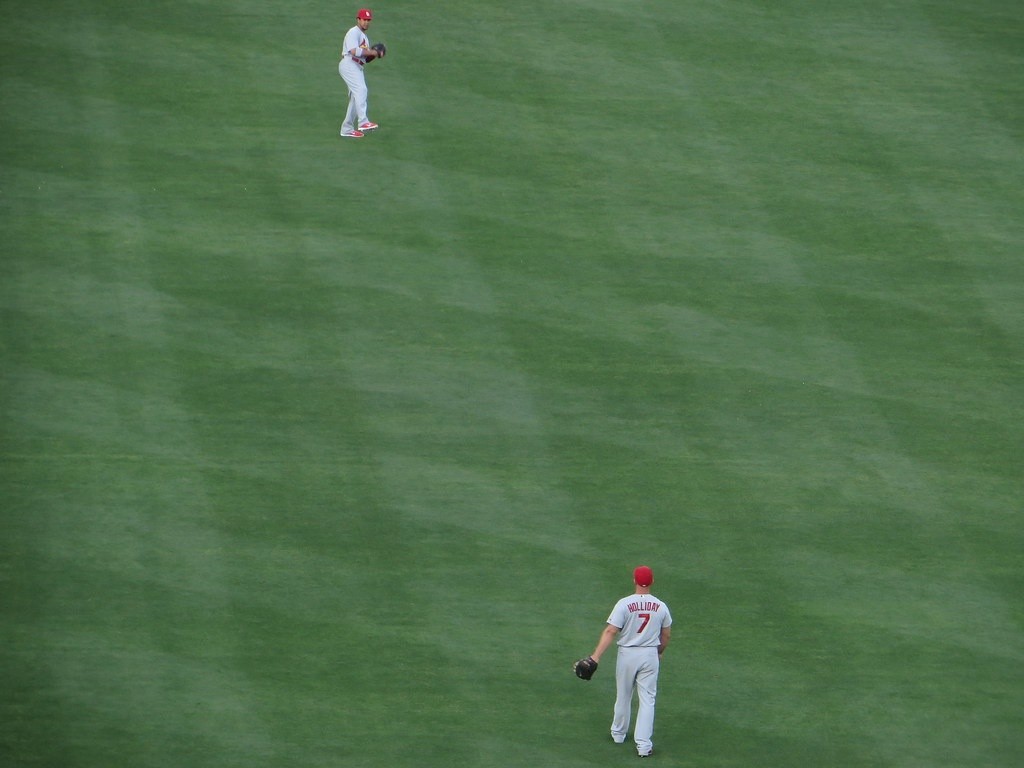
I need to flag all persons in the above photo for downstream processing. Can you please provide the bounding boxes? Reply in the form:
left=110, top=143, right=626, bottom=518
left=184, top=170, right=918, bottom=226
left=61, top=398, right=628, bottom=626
left=573, top=565, right=673, bottom=757
left=338, top=8, right=385, bottom=137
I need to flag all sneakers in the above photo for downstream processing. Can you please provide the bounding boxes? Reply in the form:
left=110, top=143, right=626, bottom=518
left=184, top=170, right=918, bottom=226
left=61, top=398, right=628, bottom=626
left=340, top=130, right=364, bottom=137
left=358, top=122, right=379, bottom=130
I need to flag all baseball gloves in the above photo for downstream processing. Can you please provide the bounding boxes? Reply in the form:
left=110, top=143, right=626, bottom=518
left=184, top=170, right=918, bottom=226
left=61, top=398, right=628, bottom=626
left=372, top=43, right=385, bottom=58
left=574, top=656, right=597, bottom=682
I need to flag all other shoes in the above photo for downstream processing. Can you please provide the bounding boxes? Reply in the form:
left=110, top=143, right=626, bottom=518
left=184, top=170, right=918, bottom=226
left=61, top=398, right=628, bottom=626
left=638, top=748, right=654, bottom=756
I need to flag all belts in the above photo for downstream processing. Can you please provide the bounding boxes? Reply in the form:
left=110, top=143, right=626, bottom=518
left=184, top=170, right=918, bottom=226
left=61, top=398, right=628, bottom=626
left=352, top=57, right=364, bottom=65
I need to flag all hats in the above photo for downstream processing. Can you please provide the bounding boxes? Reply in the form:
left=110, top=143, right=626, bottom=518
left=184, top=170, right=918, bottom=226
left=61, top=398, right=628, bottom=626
left=634, top=566, right=653, bottom=587
left=357, top=9, right=372, bottom=20
